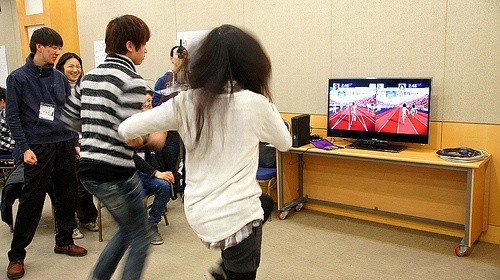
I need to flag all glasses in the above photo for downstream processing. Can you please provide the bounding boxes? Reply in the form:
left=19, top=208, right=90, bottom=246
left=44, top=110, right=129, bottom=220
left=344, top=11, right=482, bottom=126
left=49, top=45, right=63, bottom=51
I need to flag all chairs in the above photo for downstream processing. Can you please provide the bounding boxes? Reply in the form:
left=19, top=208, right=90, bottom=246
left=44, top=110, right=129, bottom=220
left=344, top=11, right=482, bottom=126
left=0, top=118, right=291, bottom=243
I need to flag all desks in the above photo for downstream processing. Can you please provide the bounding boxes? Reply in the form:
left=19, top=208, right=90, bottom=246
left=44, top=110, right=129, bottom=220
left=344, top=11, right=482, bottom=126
left=279, top=140, right=492, bottom=256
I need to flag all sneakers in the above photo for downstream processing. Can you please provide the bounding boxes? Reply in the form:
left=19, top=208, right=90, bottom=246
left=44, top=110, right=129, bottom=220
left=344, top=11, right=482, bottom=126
left=7, top=260, right=24, bottom=279
left=72, top=228, right=83, bottom=239
left=83, top=222, right=99, bottom=232
left=54, top=243, right=87, bottom=257
left=146, top=221, right=163, bottom=245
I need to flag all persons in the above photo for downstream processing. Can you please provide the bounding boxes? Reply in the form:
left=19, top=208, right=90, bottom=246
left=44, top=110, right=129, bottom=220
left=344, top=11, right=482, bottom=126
left=117, top=24, right=292, bottom=280
left=55, top=52, right=100, bottom=239
left=59, top=15, right=149, bottom=280
left=143, top=90, right=153, bottom=111
left=5, top=27, right=88, bottom=278
left=152, top=45, right=189, bottom=200
left=132, top=153, right=175, bottom=245
left=0, top=87, right=16, bottom=157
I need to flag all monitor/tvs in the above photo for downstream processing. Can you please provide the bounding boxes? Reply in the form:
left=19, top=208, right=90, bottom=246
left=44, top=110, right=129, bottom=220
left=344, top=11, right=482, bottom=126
left=326, top=77, right=433, bottom=153
